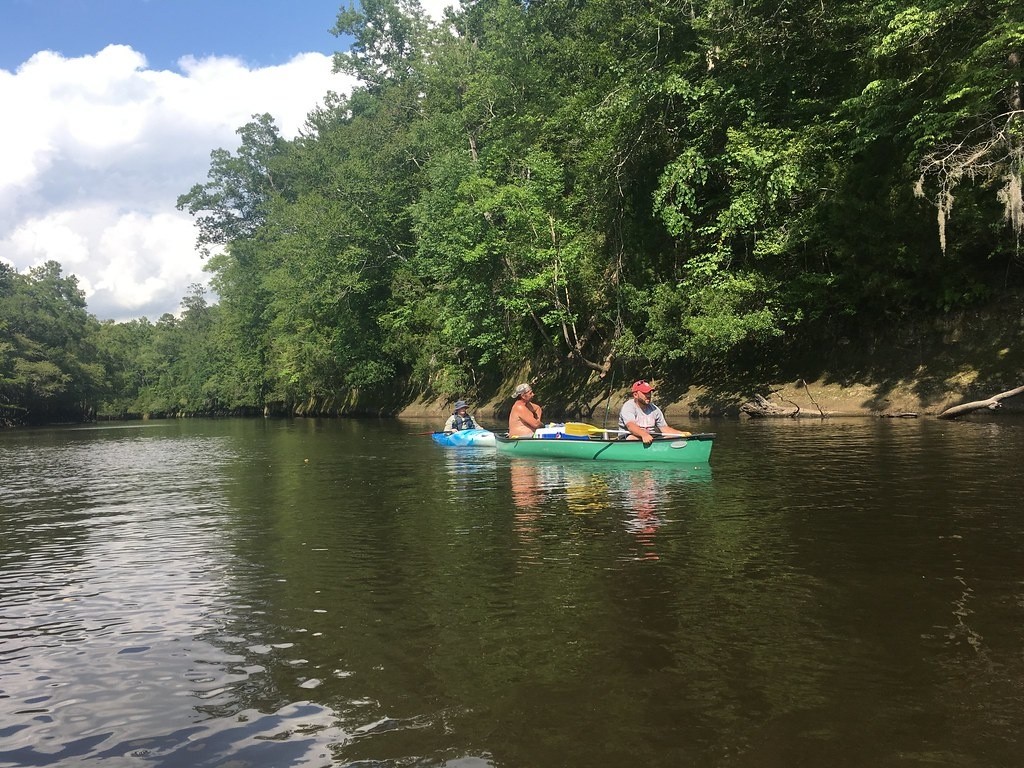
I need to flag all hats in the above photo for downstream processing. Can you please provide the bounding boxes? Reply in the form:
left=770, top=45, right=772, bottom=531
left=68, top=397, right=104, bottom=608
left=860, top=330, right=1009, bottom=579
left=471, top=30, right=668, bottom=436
left=454, top=401, right=469, bottom=410
left=510, top=383, right=530, bottom=399
left=632, top=379, right=655, bottom=393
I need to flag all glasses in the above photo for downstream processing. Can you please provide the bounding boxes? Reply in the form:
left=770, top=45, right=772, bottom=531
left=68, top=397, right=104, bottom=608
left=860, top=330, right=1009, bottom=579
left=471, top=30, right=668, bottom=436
left=637, top=379, right=647, bottom=388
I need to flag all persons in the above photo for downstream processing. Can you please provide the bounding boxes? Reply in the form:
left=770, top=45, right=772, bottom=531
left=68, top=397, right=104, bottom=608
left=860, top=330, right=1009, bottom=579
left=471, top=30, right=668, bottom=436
left=509, top=383, right=545, bottom=439
left=444, top=401, right=476, bottom=433
left=618, top=379, right=691, bottom=444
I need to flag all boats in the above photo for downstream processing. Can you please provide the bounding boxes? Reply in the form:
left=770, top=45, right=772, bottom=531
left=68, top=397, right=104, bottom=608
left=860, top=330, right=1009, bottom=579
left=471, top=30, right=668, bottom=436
left=495, top=422, right=717, bottom=463
left=432, top=429, right=496, bottom=446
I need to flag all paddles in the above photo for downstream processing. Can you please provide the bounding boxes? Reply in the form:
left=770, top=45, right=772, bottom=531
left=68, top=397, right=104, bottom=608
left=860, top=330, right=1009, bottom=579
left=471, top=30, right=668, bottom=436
left=564, top=422, right=687, bottom=437
left=408, top=427, right=509, bottom=436
